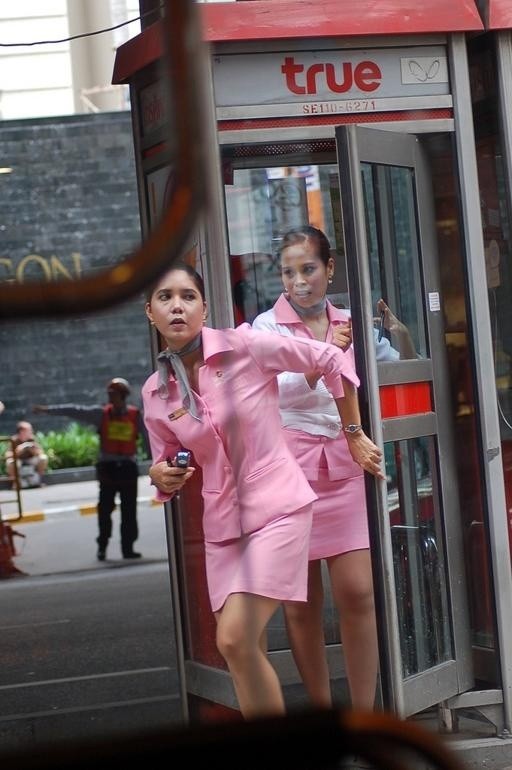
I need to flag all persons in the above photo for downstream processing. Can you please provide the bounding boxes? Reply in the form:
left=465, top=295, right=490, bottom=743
left=138, top=269, right=382, bottom=723
left=246, top=229, right=432, bottom=713
left=5, top=417, right=50, bottom=491
left=31, top=376, right=154, bottom=565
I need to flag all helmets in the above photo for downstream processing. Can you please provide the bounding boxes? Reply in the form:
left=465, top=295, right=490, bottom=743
left=104, top=377, right=131, bottom=396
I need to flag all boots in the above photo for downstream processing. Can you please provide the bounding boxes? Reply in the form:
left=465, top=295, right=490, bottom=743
left=120, top=539, right=142, bottom=560
left=93, top=537, right=108, bottom=562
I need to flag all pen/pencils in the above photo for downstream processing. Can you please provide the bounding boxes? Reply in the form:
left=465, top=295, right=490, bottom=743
left=166, top=456, right=174, bottom=467
left=378, top=310, right=384, bottom=343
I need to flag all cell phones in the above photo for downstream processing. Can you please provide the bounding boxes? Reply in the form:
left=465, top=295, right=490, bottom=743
left=176, top=450, right=192, bottom=469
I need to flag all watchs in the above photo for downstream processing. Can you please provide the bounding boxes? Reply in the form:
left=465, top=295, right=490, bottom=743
left=340, top=424, right=363, bottom=436
